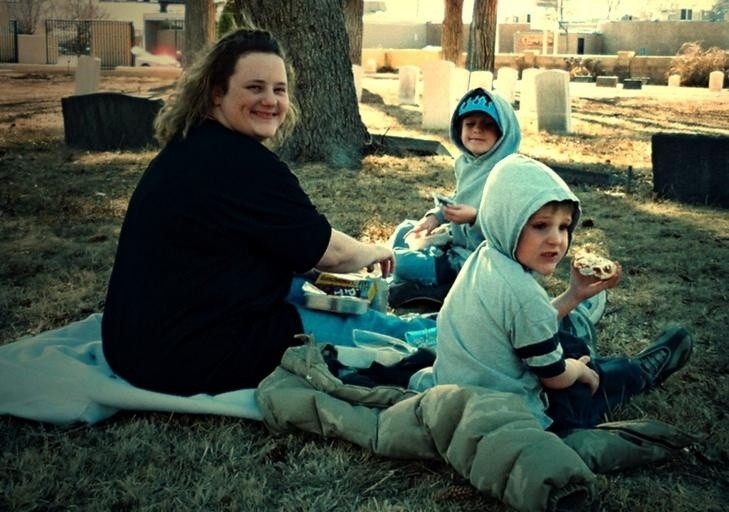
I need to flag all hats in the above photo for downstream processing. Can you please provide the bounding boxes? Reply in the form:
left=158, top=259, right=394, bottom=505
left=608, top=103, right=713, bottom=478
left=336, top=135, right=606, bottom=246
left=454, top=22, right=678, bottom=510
left=457, top=91, right=501, bottom=129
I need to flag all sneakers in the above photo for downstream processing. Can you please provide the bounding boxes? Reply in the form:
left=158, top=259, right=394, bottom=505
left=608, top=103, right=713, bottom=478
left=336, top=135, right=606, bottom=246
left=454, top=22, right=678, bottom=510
left=579, top=290, right=608, bottom=323
left=628, top=326, right=693, bottom=391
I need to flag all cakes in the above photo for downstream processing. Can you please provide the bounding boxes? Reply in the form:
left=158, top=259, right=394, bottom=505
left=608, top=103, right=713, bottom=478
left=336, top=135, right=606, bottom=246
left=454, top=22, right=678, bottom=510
left=573, top=250, right=615, bottom=279
left=404, top=225, right=449, bottom=250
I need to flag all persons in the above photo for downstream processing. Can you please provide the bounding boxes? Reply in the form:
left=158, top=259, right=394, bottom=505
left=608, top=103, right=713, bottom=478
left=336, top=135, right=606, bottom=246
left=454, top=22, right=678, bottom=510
left=379, top=86, right=521, bottom=312
left=563, top=54, right=605, bottom=82
left=430, top=151, right=692, bottom=434
left=101, top=25, right=395, bottom=397
